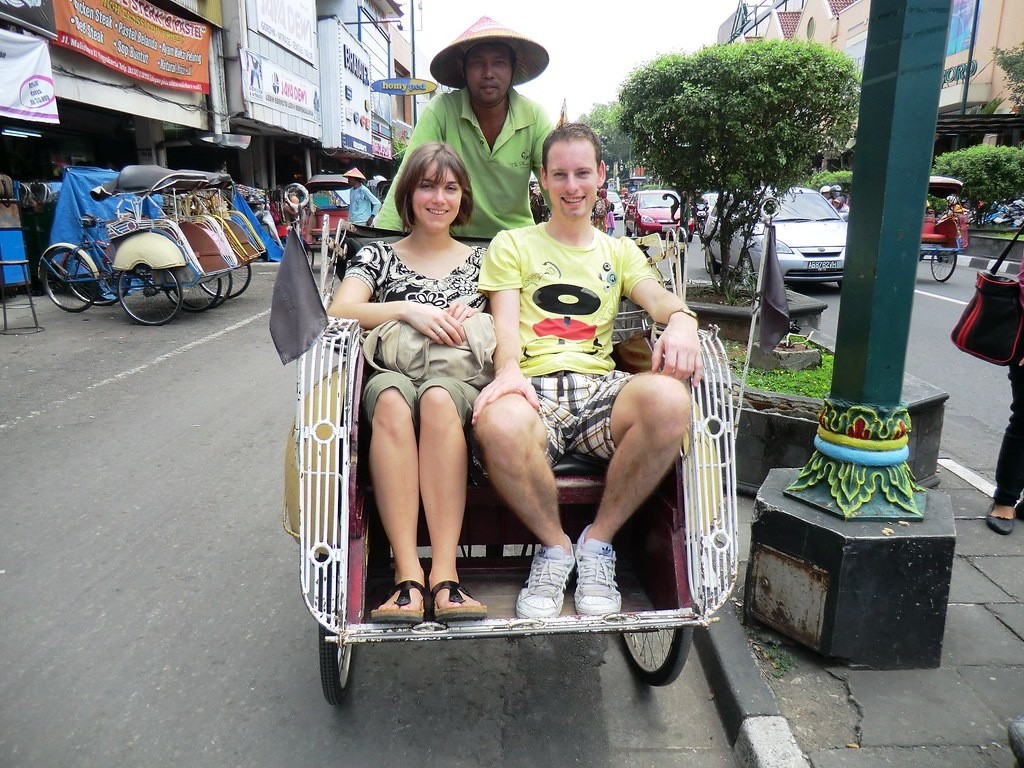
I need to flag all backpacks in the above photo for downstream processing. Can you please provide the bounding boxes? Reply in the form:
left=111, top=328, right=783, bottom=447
left=364, top=308, right=499, bottom=390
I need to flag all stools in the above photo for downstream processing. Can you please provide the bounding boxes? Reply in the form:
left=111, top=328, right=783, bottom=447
left=1, top=260, right=45, bottom=335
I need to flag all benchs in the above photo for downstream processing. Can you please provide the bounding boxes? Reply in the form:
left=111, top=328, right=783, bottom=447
left=343, top=236, right=678, bottom=546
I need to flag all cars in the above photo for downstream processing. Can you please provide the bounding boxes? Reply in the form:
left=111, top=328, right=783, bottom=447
left=606, top=192, right=625, bottom=219
left=700, top=192, right=721, bottom=225
left=624, top=190, right=694, bottom=242
left=701, top=185, right=848, bottom=288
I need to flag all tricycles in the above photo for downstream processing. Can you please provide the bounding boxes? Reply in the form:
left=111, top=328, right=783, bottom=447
left=294, top=174, right=357, bottom=269
left=38, top=164, right=263, bottom=326
left=281, top=220, right=741, bottom=711
left=917, top=175, right=965, bottom=282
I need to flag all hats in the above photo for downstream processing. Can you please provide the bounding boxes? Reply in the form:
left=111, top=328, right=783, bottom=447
left=430, top=17, right=549, bottom=90
left=342, top=166, right=366, bottom=180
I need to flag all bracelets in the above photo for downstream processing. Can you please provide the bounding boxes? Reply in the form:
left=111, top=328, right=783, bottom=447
left=371, top=215, right=374, bottom=218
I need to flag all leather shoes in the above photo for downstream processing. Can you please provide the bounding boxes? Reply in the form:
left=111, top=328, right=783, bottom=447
left=985, top=504, right=1014, bottom=535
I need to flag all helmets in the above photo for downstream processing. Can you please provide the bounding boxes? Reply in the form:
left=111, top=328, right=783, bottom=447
left=830, top=185, right=842, bottom=193
left=820, top=186, right=831, bottom=194
left=947, top=196, right=957, bottom=205
left=695, top=188, right=701, bottom=194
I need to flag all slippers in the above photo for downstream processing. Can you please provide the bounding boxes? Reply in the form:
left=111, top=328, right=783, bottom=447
left=431, top=580, right=488, bottom=623
left=370, top=580, right=425, bottom=623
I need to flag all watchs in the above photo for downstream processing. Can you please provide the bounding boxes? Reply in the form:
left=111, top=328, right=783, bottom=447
left=668, top=308, right=699, bottom=330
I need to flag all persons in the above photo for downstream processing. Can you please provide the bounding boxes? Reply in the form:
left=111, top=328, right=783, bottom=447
left=371, top=16, right=552, bottom=238
left=926, top=200, right=934, bottom=213
left=249, top=56, right=261, bottom=90
left=691, top=189, right=709, bottom=217
left=820, top=184, right=849, bottom=213
left=986, top=256, right=1024, bottom=535
left=327, top=141, right=495, bottom=623
left=591, top=189, right=615, bottom=236
left=529, top=186, right=550, bottom=224
left=471, top=123, right=701, bottom=619
left=342, top=167, right=381, bottom=227
left=940, top=196, right=964, bottom=221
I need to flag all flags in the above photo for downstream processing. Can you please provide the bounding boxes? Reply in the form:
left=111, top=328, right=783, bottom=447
left=759, top=225, right=790, bottom=355
left=269, top=220, right=330, bottom=365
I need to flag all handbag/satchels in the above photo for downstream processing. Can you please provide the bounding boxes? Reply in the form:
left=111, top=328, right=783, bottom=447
left=952, top=270, right=1024, bottom=367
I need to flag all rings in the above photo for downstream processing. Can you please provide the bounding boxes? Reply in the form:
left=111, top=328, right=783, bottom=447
left=436, top=328, right=443, bottom=335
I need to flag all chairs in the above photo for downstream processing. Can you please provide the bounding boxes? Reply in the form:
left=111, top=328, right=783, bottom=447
left=107, top=215, right=266, bottom=275
left=309, top=209, right=349, bottom=248
left=922, top=218, right=960, bottom=251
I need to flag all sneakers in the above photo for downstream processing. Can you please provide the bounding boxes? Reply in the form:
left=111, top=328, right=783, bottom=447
left=515, top=535, right=575, bottom=620
left=574, top=524, right=621, bottom=618
